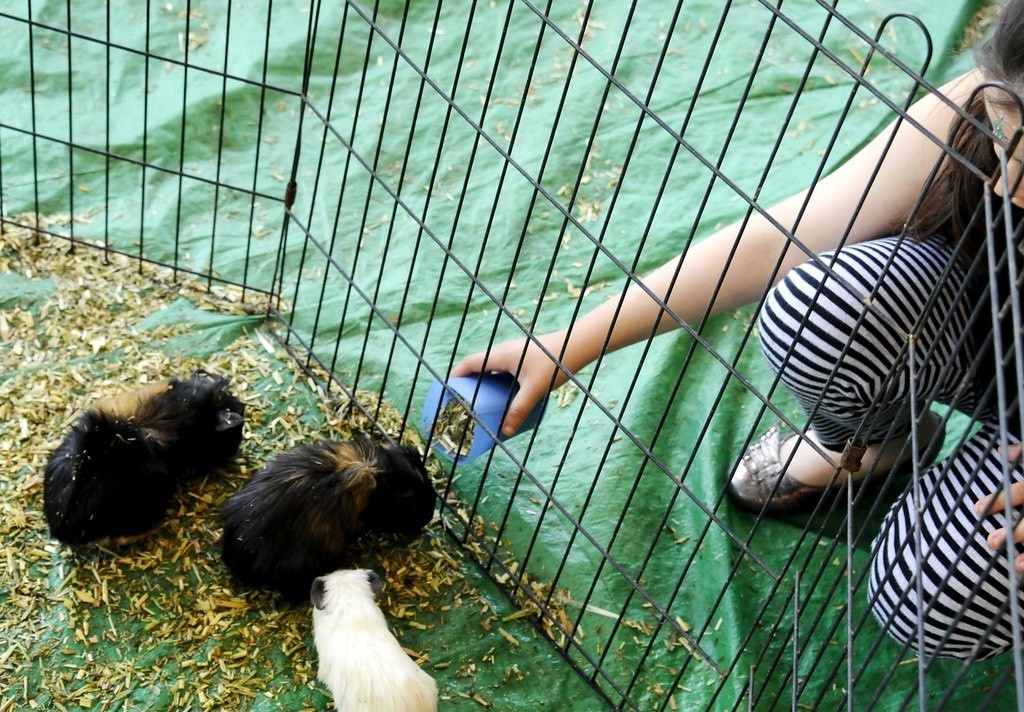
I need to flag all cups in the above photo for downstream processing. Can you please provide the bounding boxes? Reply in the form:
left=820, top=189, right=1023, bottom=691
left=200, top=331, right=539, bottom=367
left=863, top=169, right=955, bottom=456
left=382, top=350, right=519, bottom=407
left=423, top=372, right=543, bottom=467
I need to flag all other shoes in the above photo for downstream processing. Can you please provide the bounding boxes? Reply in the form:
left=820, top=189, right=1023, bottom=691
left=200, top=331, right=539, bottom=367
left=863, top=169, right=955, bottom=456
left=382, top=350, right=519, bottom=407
left=731, top=413, right=953, bottom=507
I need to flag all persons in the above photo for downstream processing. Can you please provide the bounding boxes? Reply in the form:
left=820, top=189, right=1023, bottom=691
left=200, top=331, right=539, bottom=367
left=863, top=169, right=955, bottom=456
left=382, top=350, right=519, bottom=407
left=437, top=0, right=1023, bottom=668
